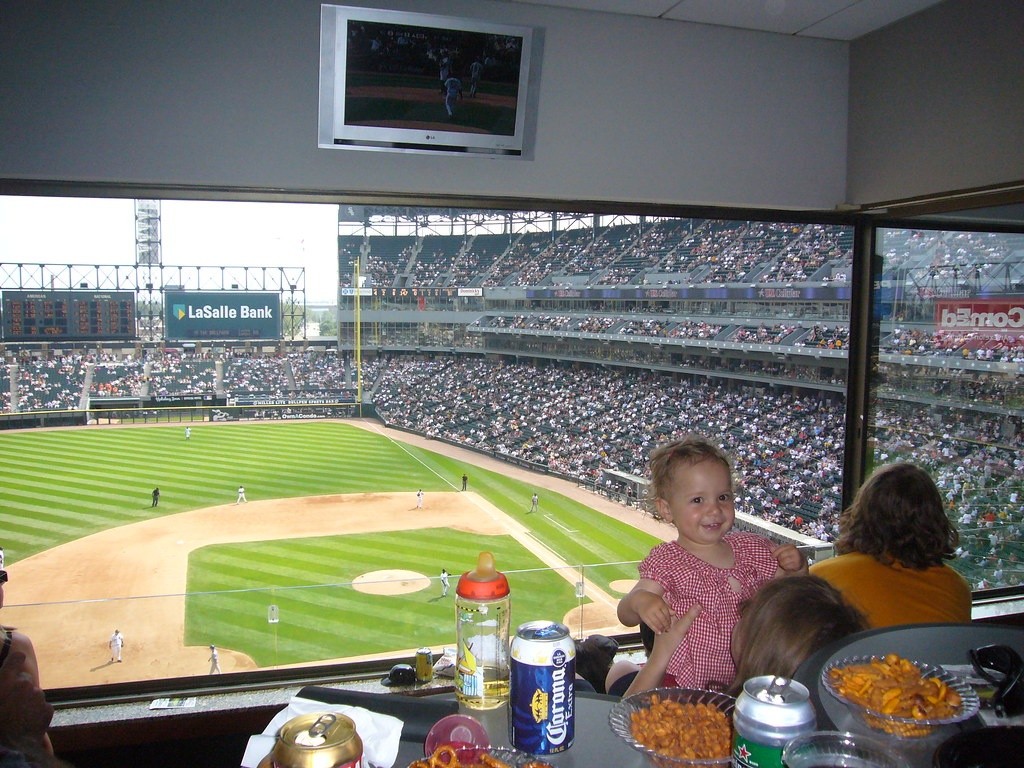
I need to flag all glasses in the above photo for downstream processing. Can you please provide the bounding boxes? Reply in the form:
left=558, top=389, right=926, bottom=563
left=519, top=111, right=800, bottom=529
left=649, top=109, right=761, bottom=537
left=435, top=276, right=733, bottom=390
left=968, top=643, right=1024, bottom=718
left=0, top=571, right=8, bottom=584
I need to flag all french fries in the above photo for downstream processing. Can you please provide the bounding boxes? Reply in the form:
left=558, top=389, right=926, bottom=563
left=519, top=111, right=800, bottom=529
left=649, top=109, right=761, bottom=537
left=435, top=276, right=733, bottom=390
left=830, top=654, right=961, bottom=736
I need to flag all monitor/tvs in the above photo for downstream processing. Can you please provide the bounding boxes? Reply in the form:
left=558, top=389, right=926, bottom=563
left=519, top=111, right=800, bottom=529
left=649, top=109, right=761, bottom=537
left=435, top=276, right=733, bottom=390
left=316, top=5, right=533, bottom=158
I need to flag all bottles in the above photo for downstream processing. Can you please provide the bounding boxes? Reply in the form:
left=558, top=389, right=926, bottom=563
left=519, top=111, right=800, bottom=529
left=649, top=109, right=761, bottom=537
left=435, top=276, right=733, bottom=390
left=454, top=552, right=511, bottom=713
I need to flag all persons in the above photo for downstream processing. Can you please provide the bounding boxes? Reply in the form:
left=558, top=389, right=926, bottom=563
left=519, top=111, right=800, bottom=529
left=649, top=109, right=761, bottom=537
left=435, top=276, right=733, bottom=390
left=185, top=427, right=192, bottom=440
left=208, top=645, right=220, bottom=675
left=606, top=575, right=872, bottom=731
left=108, top=629, right=124, bottom=663
left=0, top=345, right=337, bottom=412
left=416, top=489, right=424, bottom=510
left=339, top=217, right=1023, bottom=592
left=808, top=462, right=972, bottom=629
left=528, top=491, right=539, bottom=514
left=0, top=652, right=55, bottom=768
left=440, top=568, right=451, bottom=598
left=237, top=485, right=248, bottom=505
left=152, top=488, right=160, bottom=507
left=0, top=547, right=5, bottom=571
left=0, top=569, right=55, bottom=768
left=212, top=409, right=226, bottom=421
left=461, top=473, right=468, bottom=492
left=617, top=434, right=810, bottom=691
left=575, top=634, right=618, bottom=694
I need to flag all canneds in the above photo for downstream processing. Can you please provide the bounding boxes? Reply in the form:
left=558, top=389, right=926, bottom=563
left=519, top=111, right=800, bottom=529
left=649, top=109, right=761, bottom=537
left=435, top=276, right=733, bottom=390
left=731, top=676, right=816, bottom=768
left=415, top=647, right=435, bottom=684
left=507, top=620, right=576, bottom=756
left=271, top=711, right=363, bottom=768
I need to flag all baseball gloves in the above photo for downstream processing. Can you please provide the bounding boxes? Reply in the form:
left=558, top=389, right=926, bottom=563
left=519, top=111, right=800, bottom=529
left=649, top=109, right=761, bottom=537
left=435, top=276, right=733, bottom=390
left=448, top=573, right=451, bottom=576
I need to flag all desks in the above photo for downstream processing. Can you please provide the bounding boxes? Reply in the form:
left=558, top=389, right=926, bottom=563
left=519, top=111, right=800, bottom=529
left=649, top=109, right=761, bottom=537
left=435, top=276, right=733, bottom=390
left=789, top=623, right=1024, bottom=768
left=378, top=688, right=659, bottom=768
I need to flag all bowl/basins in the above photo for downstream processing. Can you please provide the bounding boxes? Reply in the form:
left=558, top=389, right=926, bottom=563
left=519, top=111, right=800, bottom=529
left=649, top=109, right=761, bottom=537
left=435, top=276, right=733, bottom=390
left=405, top=747, right=556, bottom=767
left=822, top=655, right=981, bottom=741
left=783, top=729, right=915, bottom=768
left=608, top=687, right=737, bottom=768
